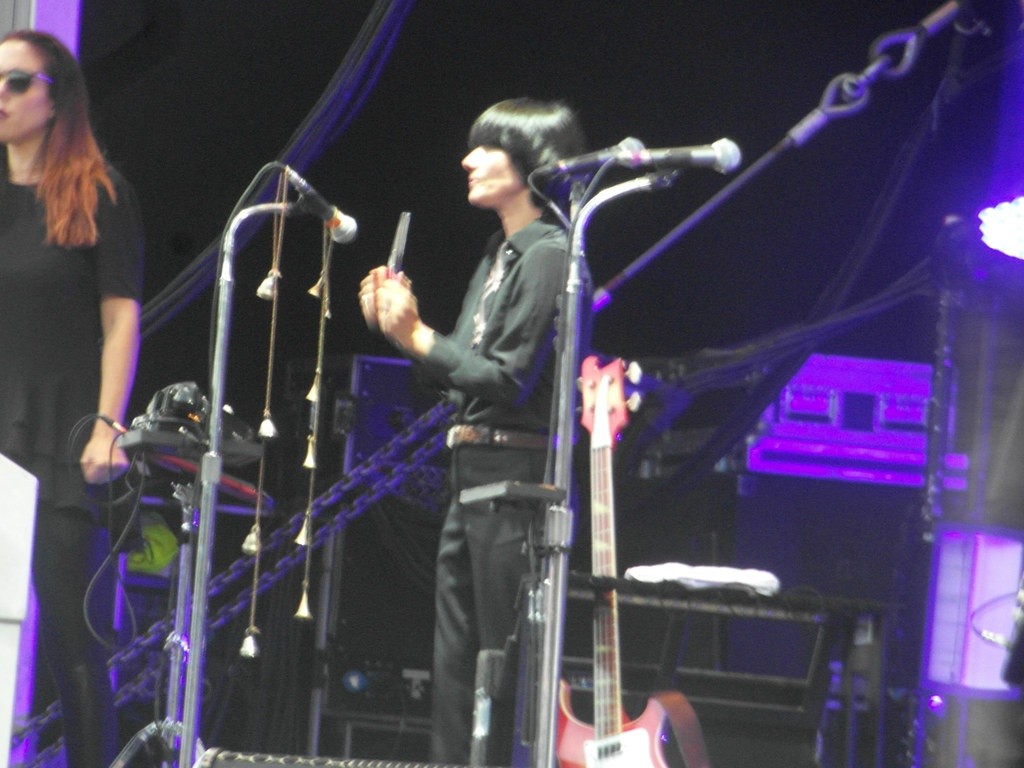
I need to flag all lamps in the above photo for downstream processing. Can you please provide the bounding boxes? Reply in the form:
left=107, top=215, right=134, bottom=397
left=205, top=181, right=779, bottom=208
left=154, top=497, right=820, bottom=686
left=935, top=189, right=1024, bottom=292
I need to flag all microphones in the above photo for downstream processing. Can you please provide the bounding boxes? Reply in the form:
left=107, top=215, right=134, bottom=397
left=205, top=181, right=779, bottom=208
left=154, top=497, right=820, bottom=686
left=280, top=164, right=357, bottom=246
left=551, top=134, right=741, bottom=176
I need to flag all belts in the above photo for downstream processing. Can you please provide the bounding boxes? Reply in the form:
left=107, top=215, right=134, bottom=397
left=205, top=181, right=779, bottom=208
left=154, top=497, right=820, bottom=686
left=445, top=425, right=549, bottom=450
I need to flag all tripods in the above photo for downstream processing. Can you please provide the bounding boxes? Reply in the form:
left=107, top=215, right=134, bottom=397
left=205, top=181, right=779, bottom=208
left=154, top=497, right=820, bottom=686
left=109, top=483, right=215, bottom=768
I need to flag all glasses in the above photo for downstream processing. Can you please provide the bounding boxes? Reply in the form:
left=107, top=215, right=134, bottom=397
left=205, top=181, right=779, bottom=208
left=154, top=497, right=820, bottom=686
left=0, top=70, right=61, bottom=94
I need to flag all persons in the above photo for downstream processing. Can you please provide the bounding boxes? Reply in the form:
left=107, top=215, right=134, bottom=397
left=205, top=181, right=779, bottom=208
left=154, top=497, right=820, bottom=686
left=0, top=29, right=143, bottom=767
left=357, top=98, right=596, bottom=767
